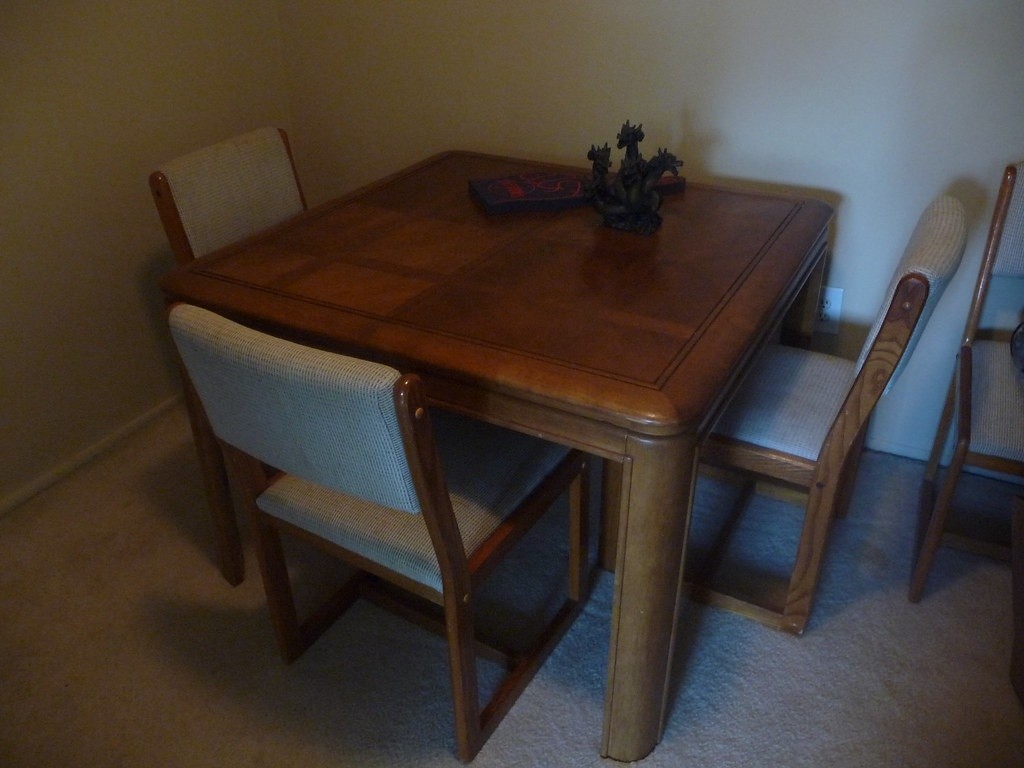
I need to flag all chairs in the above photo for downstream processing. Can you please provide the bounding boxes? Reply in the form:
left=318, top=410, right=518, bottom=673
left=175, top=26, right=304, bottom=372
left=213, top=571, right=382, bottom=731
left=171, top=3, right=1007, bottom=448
left=912, top=163, right=1024, bottom=609
left=598, top=193, right=964, bottom=636
left=164, top=300, right=590, bottom=764
left=152, top=122, right=310, bottom=267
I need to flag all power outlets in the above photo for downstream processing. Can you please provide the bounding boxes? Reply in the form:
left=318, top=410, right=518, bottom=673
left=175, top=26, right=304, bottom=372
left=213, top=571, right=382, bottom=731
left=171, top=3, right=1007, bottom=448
left=814, top=286, right=842, bottom=335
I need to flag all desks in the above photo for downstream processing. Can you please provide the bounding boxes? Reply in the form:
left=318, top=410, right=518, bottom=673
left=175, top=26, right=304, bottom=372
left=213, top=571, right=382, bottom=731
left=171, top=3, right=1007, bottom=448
left=158, top=148, right=831, bottom=759
